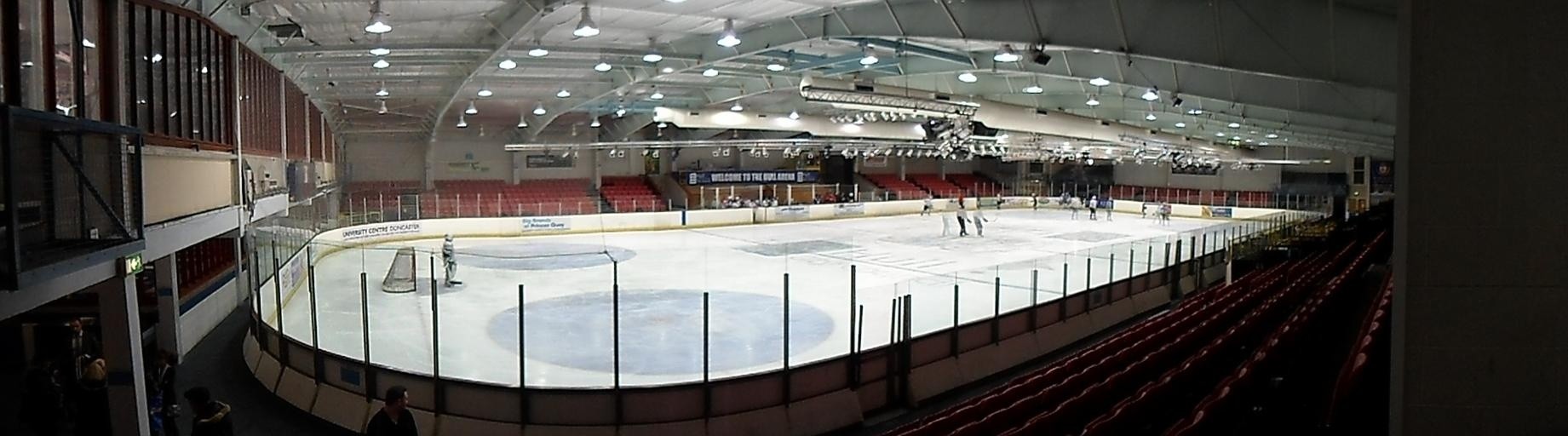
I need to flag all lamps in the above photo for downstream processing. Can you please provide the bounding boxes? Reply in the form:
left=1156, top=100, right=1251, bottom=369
left=714, top=19, right=743, bottom=49
left=857, top=45, right=883, bottom=68
left=452, top=43, right=569, bottom=138
left=364, top=1, right=393, bottom=116
left=590, top=52, right=717, bottom=140
left=991, top=42, right=1022, bottom=66
left=958, top=70, right=1046, bottom=99
left=728, top=60, right=804, bottom=122
left=570, top=6, right=606, bottom=40
left=1082, top=76, right=1277, bottom=153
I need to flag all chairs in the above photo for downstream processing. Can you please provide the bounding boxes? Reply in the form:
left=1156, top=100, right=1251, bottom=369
left=883, top=229, right=1393, bottom=436
left=863, top=172, right=1002, bottom=200
left=340, top=175, right=667, bottom=218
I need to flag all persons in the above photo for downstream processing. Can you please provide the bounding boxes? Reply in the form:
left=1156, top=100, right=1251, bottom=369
left=366, top=385, right=419, bottom=436
left=997, top=189, right=1002, bottom=209
left=1031, top=191, right=1038, bottom=210
left=1105, top=197, right=1114, bottom=222
left=920, top=195, right=934, bottom=216
left=51, top=315, right=235, bottom=435
left=1089, top=195, right=1098, bottom=221
left=1155, top=203, right=1171, bottom=226
left=1057, top=192, right=1087, bottom=212
left=977, top=192, right=982, bottom=210
left=957, top=206, right=972, bottom=236
left=1069, top=194, right=1081, bottom=220
left=941, top=200, right=954, bottom=236
left=958, top=191, right=963, bottom=207
left=973, top=207, right=989, bottom=238
left=443, top=233, right=457, bottom=286
left=711, top=191, right=855, bottom=208
